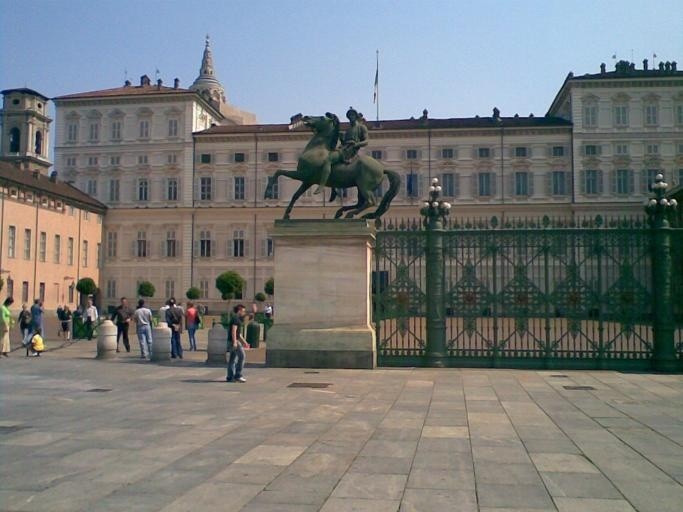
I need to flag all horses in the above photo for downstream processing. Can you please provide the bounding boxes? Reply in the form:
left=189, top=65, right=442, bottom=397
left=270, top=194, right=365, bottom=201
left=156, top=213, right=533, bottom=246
left=263, top=112, right=401, bottom=220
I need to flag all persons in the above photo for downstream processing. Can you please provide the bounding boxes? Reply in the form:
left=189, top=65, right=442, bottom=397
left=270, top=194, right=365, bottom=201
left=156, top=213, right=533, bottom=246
left=56, top=300, right=98, bottom=341
left=226, top=304, right=250, bottom=383
left=111, top=297, right=134, bottom=352
left=0, top=297, right=45, bottom=358
left=313, top=106, right=368, bottom=202
left=265, top=303, right=272, bottom=319
left=158, top=297, right=197, bottom=358
left=132, top=299, right=153, bottom=361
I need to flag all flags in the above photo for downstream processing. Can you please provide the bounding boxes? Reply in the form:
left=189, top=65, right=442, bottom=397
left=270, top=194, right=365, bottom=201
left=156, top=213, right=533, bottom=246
left=374, top=69, right=378, bottom=104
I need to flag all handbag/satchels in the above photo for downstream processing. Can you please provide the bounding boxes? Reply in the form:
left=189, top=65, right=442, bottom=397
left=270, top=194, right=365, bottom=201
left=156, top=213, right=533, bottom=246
left=195, top=315, right=200, bottom=325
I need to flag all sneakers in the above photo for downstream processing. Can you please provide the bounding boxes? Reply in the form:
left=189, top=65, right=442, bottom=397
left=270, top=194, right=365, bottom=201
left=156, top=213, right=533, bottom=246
left=226, top=377, right=246, bottom=382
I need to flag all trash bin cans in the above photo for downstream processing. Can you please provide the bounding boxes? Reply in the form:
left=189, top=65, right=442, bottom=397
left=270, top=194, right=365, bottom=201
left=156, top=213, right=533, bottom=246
left=73, top=317, right=89, bottom=339
left=254, top=312, right=264, bottom=323
left=247, top=322, right=261, bottom=348
left=153, top=315, right=160, bottom=326
